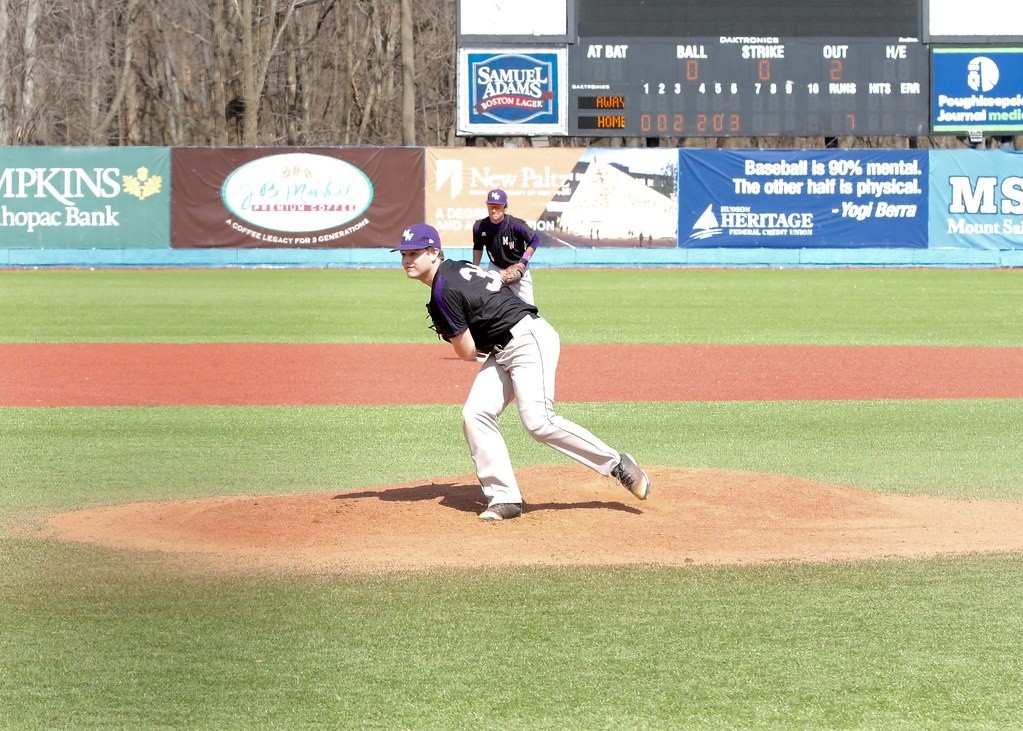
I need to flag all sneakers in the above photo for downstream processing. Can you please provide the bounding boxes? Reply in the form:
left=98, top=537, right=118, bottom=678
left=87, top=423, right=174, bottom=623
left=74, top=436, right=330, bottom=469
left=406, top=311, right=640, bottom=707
left=612, top=453, right=650, bottom=501
left=479, top=502, right=524, bottom=521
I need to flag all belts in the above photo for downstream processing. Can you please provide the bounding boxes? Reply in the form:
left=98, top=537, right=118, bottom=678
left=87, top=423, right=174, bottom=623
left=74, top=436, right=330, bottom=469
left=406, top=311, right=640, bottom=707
left=491, top=313, right=540, bottom=355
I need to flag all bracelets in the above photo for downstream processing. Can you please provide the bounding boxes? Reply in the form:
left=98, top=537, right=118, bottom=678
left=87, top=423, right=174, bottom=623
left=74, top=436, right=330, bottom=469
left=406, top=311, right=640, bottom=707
left=520, top=258, right=528, bottom=266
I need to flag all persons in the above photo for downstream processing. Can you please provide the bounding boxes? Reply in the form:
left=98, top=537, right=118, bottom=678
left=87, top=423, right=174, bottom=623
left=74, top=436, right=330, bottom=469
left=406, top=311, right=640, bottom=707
left=473, top=189, right=540, bottom=306
left=390, top=225, right=650, bottom=521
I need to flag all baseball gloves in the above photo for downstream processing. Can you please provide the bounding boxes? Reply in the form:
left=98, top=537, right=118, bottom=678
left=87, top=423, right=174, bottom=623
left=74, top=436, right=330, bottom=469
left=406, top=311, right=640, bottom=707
left=498, top=265, right=525, bottom=285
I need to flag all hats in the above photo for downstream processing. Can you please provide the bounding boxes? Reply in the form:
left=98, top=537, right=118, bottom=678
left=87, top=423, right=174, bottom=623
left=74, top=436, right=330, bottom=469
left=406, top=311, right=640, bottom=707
left=390, top=224, right=441, bottom=253
left=486, top=189, right=508, bottom=205
